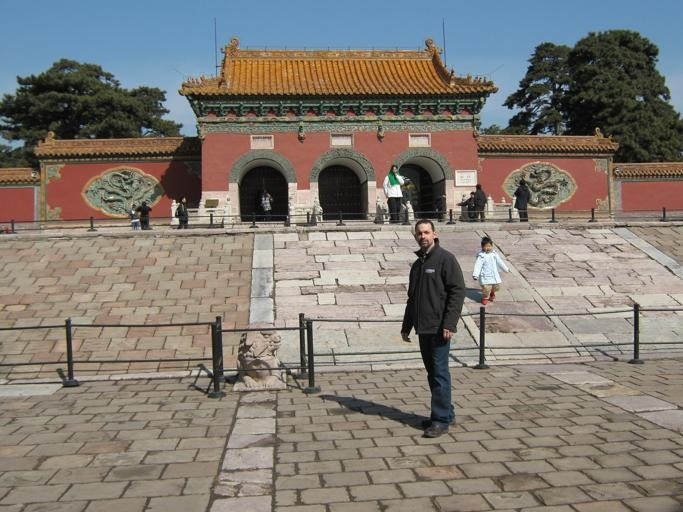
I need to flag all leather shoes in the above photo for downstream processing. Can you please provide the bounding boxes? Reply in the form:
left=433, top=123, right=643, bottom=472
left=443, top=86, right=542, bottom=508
left=422, top=416, right=456, bottom=437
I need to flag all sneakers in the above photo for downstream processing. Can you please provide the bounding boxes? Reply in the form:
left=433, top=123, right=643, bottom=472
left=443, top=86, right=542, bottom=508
left=482, top=298, right=489, bottom=304
left=490, top=292, right=495, bottom=300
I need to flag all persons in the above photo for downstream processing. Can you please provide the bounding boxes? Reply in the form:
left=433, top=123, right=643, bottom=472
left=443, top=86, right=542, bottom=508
left=514, top=180, right=530, bottom=222
left=473, top=184, right=487, bottom=221
left=401, top=220, right=464, bottom=438
left=457, top=192, right=476, bottom=221
left=260, top=188, right=274, bottom=222
left=128, top=204, right=140, bottom=230
left=472, top=237, right=509, bottom=304
left=383, top=165, right=405, bottom=223
left=432, top=194, right=446, bottom=222
left=176, top=196, right=188, bottom=229
left=136, top=202, right=152, bottom=230
